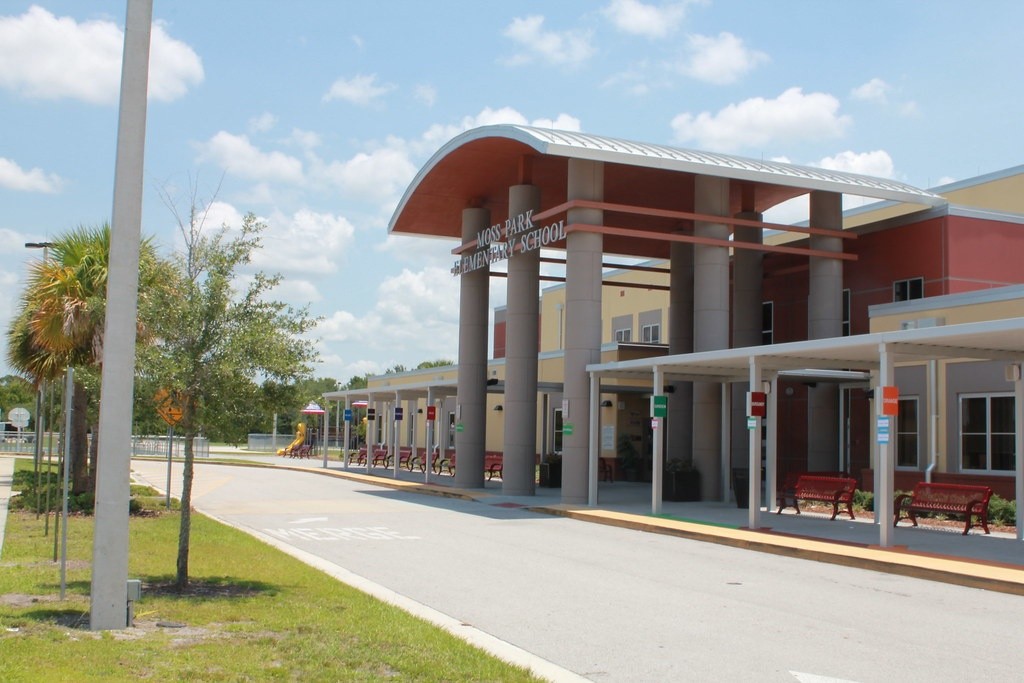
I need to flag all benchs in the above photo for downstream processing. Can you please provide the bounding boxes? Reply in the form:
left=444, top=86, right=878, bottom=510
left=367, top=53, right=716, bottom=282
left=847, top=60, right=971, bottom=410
left=409, top=452, right=438, bottom=474
left=385, top=451, right=411, bottom=470
left=894, top=481, right=994, bottom=536
left=293, top=445, right=311, bottom=459
left=363, top=449, right=387, bottom=468
left=776, top=475, right=857, bottom=521
left=349, top=448, right=367, bottom=466
left=484, top=456, right=503, bottom=481
left=283, top=445, right=301, bottom=458
left=437, top=454, right=455, bottom=477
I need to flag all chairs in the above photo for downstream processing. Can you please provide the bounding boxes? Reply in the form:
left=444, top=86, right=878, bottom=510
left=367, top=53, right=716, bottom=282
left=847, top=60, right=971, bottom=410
left=599, top=457, right=613, bottom=483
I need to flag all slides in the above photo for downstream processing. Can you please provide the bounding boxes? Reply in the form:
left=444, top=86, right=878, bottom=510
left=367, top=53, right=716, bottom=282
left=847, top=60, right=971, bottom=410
left=277, top=437, right=305, bottom=456
